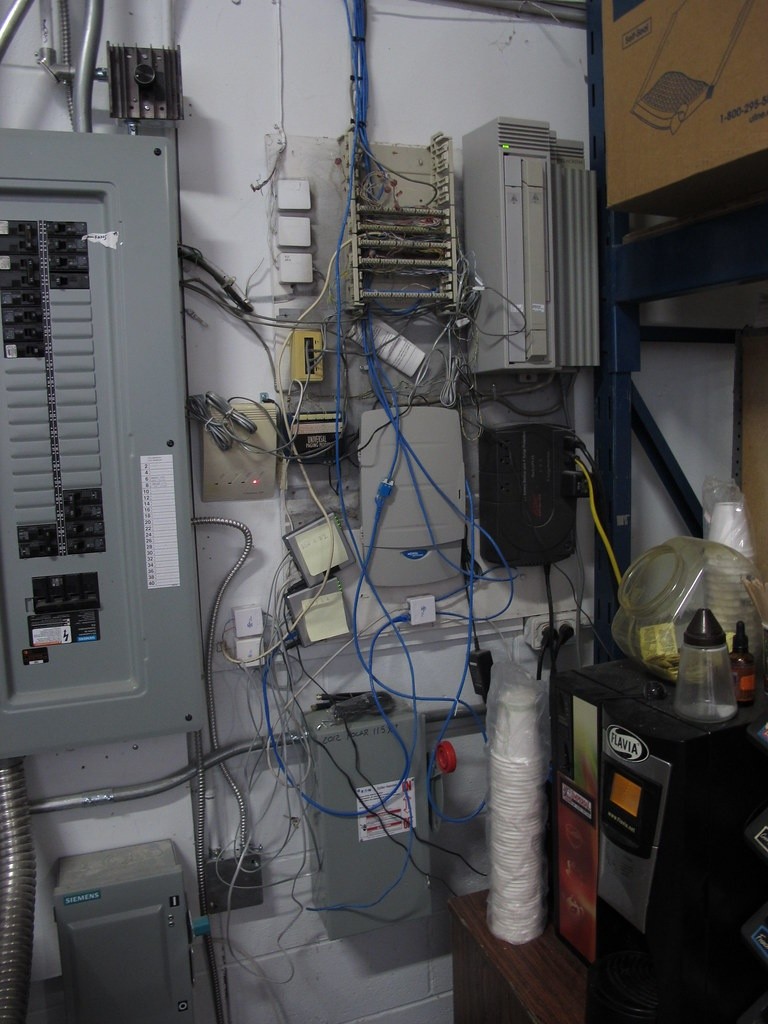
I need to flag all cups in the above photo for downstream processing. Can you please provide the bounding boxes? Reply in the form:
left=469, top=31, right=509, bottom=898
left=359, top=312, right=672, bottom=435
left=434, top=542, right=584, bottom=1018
left=705, top=500, right=755, bottom=653
left=489, top=688, right=545, bottom=945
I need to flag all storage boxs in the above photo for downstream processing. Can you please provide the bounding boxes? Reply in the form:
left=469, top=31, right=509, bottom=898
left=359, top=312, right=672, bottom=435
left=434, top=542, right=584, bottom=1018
left=599, top=0, right=768, bottom=221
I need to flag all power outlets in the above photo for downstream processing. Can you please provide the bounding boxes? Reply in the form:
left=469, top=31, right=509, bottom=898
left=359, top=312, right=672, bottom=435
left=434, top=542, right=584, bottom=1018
left=524, top=611, right=578, bottom=650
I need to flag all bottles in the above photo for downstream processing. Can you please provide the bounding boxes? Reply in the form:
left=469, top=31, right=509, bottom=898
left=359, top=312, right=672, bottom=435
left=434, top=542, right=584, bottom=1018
left=611, top=535, right=763, bottom=680
left=674, top=607, right=738, bottom=723
left=729, top=621, right=756, bottom=706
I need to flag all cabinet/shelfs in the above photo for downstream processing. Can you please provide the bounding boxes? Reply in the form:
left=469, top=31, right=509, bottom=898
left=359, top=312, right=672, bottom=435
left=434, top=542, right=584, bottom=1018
left=445, top=889, right=591, bottom=1024
